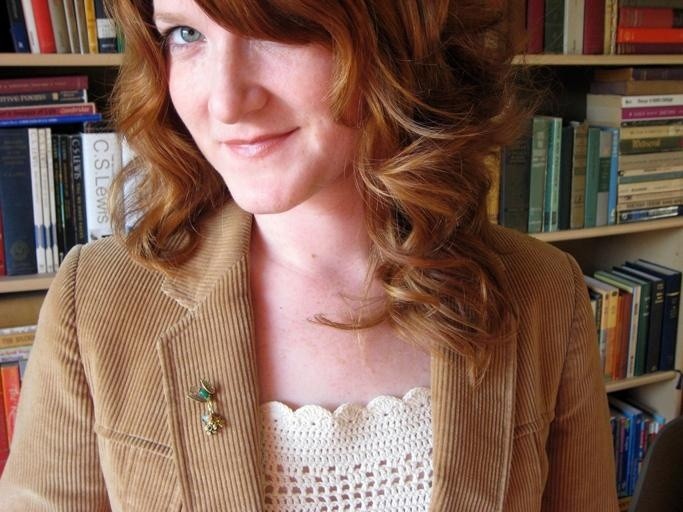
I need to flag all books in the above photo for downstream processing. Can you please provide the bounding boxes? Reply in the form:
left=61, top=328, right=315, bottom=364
left=487, top=0, right=683, bottom=54
left=2, top=65, right=150, bottom=277
left=485, top=66, right=683, bottom=233
left=1, top=0, right=133, bottom=54
left=578, top=255, right=683, bottom=384
left=2, top=326, right=37, bottom=468
left=611, top=401, right=665, bottom=501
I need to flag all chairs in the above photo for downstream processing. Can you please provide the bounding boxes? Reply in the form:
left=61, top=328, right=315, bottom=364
left=628, top=416, right=683, bottom=512
